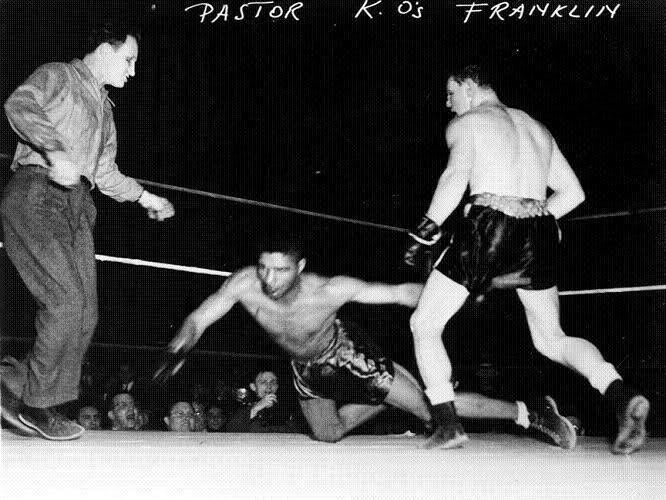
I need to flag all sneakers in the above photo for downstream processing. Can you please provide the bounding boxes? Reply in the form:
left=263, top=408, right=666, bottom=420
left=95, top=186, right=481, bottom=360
left=0, top=381, right=38, bottom=437
left=18, top=405, right=85, bottom=440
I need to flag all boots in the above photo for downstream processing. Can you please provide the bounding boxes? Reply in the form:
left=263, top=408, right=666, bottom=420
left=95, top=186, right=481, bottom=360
left=418, top=401, right=469, bottom=451
left=517, top=396, right=576, bottom=450
left=605, top=379, right=651, bottom=453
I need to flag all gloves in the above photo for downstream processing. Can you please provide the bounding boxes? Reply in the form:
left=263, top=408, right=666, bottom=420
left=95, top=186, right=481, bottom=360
left=404, top=215, right=443, bottom=267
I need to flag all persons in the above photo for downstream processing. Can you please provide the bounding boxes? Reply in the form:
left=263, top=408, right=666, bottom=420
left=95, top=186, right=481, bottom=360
left=72, top=352, right=296, bottom=433
left=0, top=18, right=175, bottom=444
left=403, top=63, right=651, bottom=454
left=153, top=231, right=575, bottom=451
